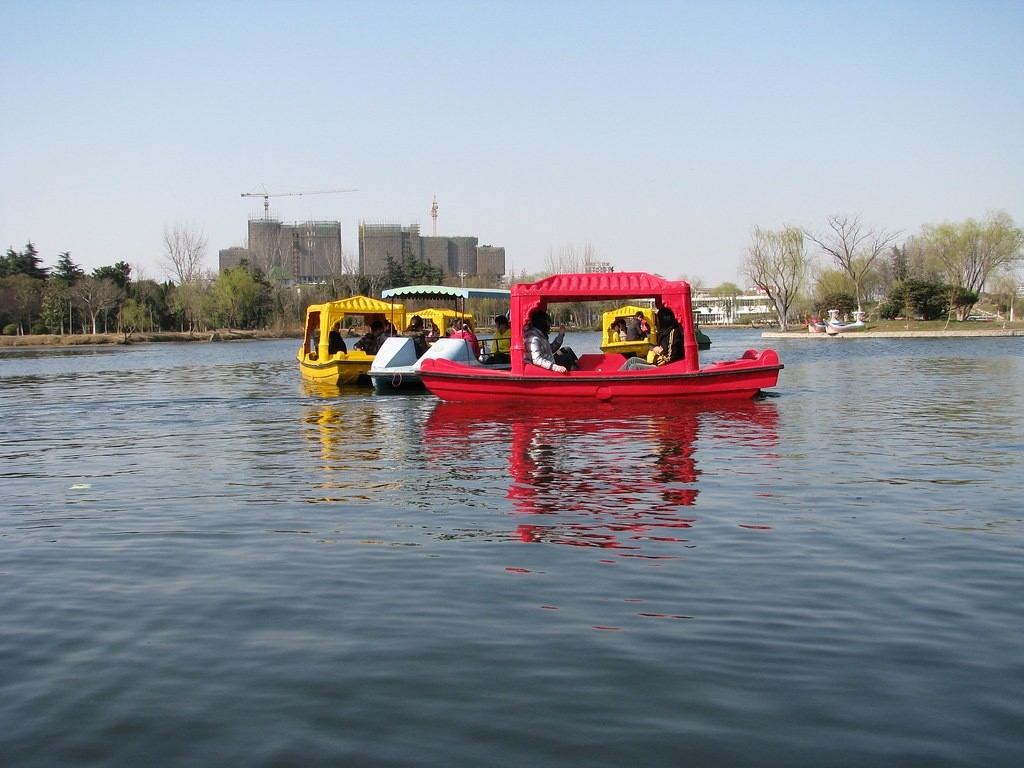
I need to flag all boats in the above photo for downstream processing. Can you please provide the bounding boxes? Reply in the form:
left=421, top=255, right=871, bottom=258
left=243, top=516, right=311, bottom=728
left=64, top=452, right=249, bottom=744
left=402, top=307, right=477, bottom=351
left=412, top=272, right=786, bottom=404
left=365, top=284, right=530, bottom=394
left=808, top=310, right=844, bottom=332
left=822, top=311, right=865, bottom=334
left=296, top=295, right=407, bottom=389
left=422, top=398, right=780, bottom=555
left=599, top=305, right=658, bottom=361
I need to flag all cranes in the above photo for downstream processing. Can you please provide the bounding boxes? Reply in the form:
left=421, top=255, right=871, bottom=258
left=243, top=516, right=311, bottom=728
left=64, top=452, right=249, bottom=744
left=238, top=183, right=361, bottom=220
left=431, top=192, right=442, bottom=237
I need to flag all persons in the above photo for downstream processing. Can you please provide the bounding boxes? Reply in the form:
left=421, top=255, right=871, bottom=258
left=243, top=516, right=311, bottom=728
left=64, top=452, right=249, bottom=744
left=352, top=320, right=397, bottom=355
left=614, top=319, right=648, bottom=338
left=618, top=307, right=685, bottom=371
left=422, top=319, right=459, bottom=341
left=484, top=315, right=511, bottom=364
left=449, top=318, right=481, bottom=358
left=523, top=311, right=579, bottom=372
left=400, top=315, right=430, bottom=359
left=328, top=323, right=347, bottom=355
left=626, top=311, right=647, bottom=341
